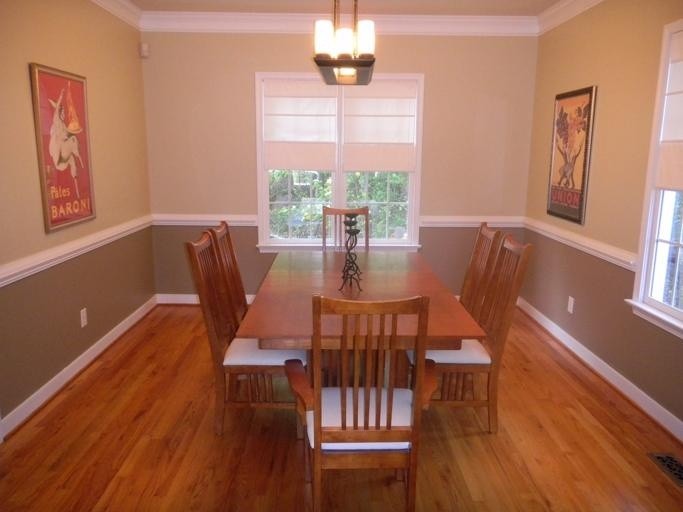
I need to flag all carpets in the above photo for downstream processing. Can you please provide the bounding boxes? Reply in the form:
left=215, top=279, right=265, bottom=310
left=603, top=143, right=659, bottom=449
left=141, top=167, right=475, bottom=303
left=648, top=451, right=683, bottom=487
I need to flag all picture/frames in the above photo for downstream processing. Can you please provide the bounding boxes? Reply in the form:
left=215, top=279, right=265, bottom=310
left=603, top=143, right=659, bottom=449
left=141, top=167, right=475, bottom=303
left=546, top=85, right=596, bottom=226
left=29, top=62, right=96, bottom=234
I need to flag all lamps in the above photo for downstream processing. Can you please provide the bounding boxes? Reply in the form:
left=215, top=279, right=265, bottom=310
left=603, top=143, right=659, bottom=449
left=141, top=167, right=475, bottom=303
left=313, top=0, right=375, bottom=85
